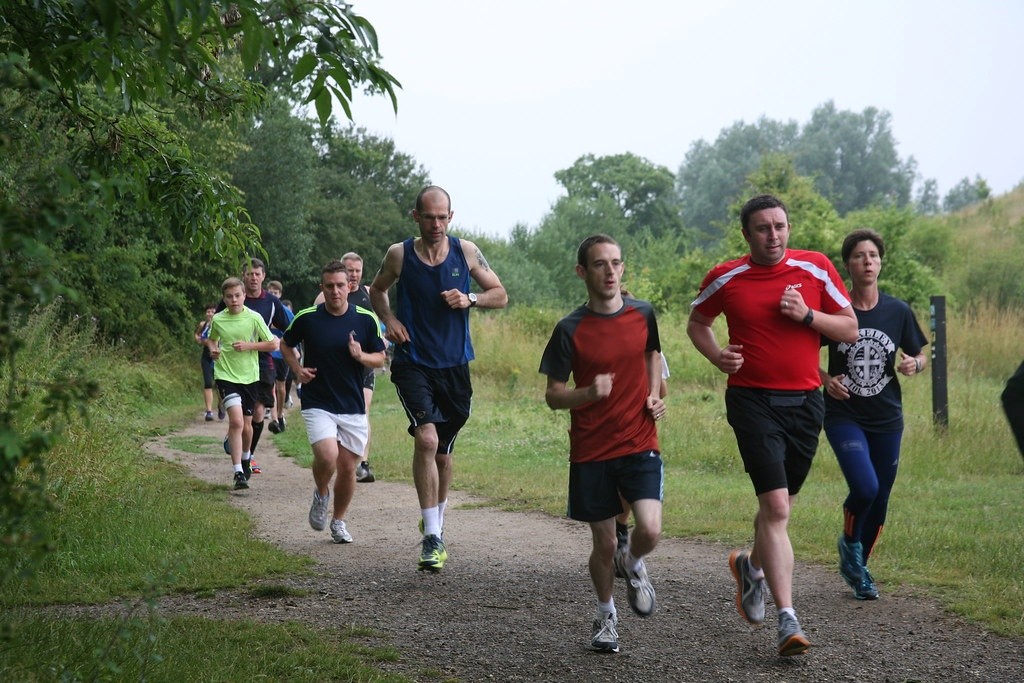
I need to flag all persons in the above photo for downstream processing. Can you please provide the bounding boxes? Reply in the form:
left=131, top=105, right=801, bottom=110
left=369, top=186, right=508, bottom=570
left=279, top=260, right=385, bottom=544
left=195, top=256, right=305, bottom=473
left=818, top=228, right=931, bottom=602
left=207, top=277, right=277, bottom=490
left=314, top=253, right=394, bottom=482
left=686, top=194, right=858, bottom=655
left=614, top=288, right=671, bottom=579
left=539, top=235, right=667, bottom=651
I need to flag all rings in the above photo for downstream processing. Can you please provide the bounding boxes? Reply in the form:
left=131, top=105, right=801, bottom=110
left=657, top=414, right=659, bottom=418
left=785, top=302, right=788, bottom=308
left=353, top=349, right=356, bottom=352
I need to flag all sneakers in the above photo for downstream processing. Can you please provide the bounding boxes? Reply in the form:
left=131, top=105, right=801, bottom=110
left=418, top=518, right=444, bottom=541
left=411, top=534, right=448, bottom=572
left=837, top=530, right=866, bottom=584
left=268, top=420, right=281, bottom=434
left=355, top=461, right=375, bottom=483
left=728, top=551, right=768, bottom=624
left=240, top=449, right=251, bottom=481
left=309, top=485, right=330, bottom=530
left=263, top=408, right=272, bottom=420
left=776, top=609, right=811, bottom=657
left=278, top=414, right=287, bottom=432
left=249, top=455, right=261, bottom=473
left=330, top=517, right=353, bottom=544
left=217, top=402, right=226, bottom=420
left=205, top=410, right=214, bottom=422
left=614, top=523, right=633, bottom=578
left=588, top=612, right=620, bottom=653
left=224, top=431, right=231, bottom=455
left=283, top=395, right=293, bottom=410
left=848, top=565, right=880, bottom=600
left=616, top=545, right=657, bottom=618
left=233, top=471, right=249, bottom=490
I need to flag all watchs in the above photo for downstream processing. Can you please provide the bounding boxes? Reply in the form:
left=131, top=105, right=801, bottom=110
left=804, top=307, right=814, bottom=325
left=915, top=359, right=922, bottom=372
left=468, top=293, right=477, bottom=306
